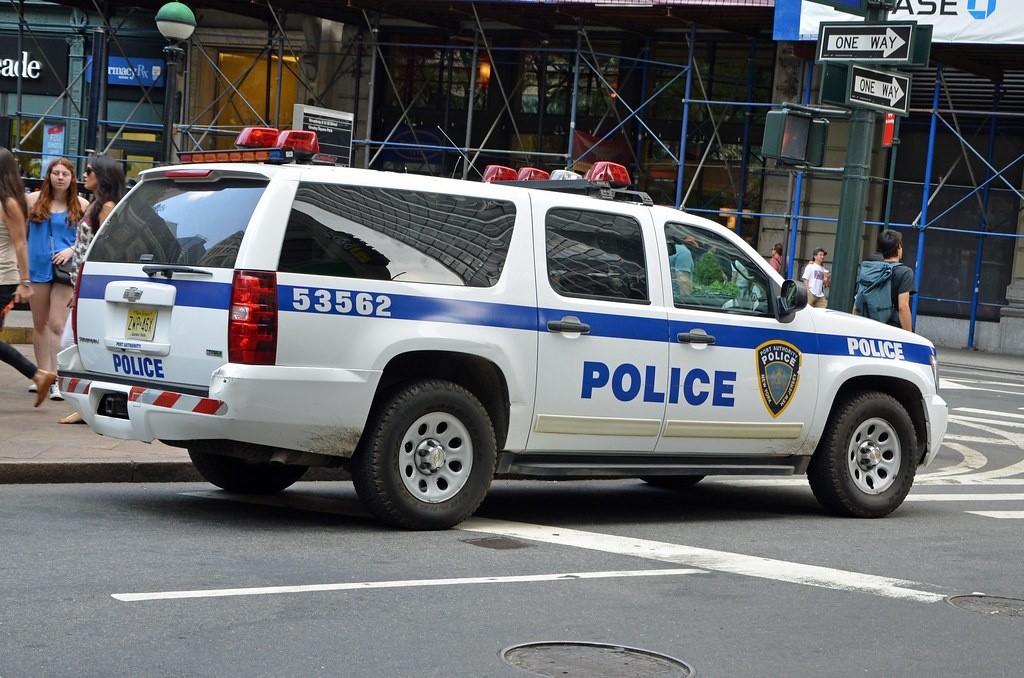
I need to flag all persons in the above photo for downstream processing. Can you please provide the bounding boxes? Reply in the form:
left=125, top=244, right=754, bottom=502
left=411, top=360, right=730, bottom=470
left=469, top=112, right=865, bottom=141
left=28, top=159, right=90, bottom=401
left=669, top=245, right=694, bottom=296
left=771, top=243, right=783, bottom=272
left=0, top=147, right=56, bottom=407
left=59, top=155, right=127, bottom=424
left=851, top=229, right=918, bottom=333
left=803, top=247, right=830, bottom=308
left=730, top=259, right=751, bottom=294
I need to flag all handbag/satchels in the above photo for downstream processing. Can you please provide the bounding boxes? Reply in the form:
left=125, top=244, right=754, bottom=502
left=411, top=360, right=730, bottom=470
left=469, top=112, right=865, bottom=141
left=53, top=260, right=73, bottom=284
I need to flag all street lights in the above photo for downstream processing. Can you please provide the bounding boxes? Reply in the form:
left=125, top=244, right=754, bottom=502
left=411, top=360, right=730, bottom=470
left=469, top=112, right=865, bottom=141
left=155, top=1, right=197, bottom=165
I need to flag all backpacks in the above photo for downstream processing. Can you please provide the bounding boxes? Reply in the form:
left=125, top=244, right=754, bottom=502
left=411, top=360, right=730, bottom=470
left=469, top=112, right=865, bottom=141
left=852, top=259, right=903, bottom=324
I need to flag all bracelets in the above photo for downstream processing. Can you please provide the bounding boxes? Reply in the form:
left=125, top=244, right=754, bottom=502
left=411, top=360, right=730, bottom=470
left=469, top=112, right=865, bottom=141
left=70, top=247, right=74, bottom=251
left=21, top=281, right=30, bottom=286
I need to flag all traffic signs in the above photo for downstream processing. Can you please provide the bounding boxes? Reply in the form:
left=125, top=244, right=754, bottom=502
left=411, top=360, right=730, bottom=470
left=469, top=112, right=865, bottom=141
left=813, top=20, right=914, bottom=117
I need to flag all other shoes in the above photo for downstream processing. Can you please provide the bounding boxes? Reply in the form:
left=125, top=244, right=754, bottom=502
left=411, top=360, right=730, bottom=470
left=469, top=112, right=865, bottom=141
left=34, top=371, right=58, bottom=408
left=49, top=380, right=65, bottom=401
left=29, top=384, right=39, bottom=392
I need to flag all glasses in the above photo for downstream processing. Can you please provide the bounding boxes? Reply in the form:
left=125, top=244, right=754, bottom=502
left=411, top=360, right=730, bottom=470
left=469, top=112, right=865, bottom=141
left=83, top=166, right=95, bottom=176
left=772, top=248, right=775, bottom=251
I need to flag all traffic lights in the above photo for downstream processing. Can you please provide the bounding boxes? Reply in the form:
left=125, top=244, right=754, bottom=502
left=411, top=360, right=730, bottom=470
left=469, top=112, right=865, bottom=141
left=805, top=119, right=828, bottom=167
left=762, top=107, right=810, bottom=164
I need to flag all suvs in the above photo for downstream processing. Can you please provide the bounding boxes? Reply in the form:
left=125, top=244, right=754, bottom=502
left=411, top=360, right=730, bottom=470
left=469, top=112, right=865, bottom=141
left=56, top=127, right=949, bottom=531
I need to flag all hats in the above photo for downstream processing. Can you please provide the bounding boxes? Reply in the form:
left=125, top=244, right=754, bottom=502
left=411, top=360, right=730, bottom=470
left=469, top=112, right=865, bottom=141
left=816, top=246, right=827, bottom=256
